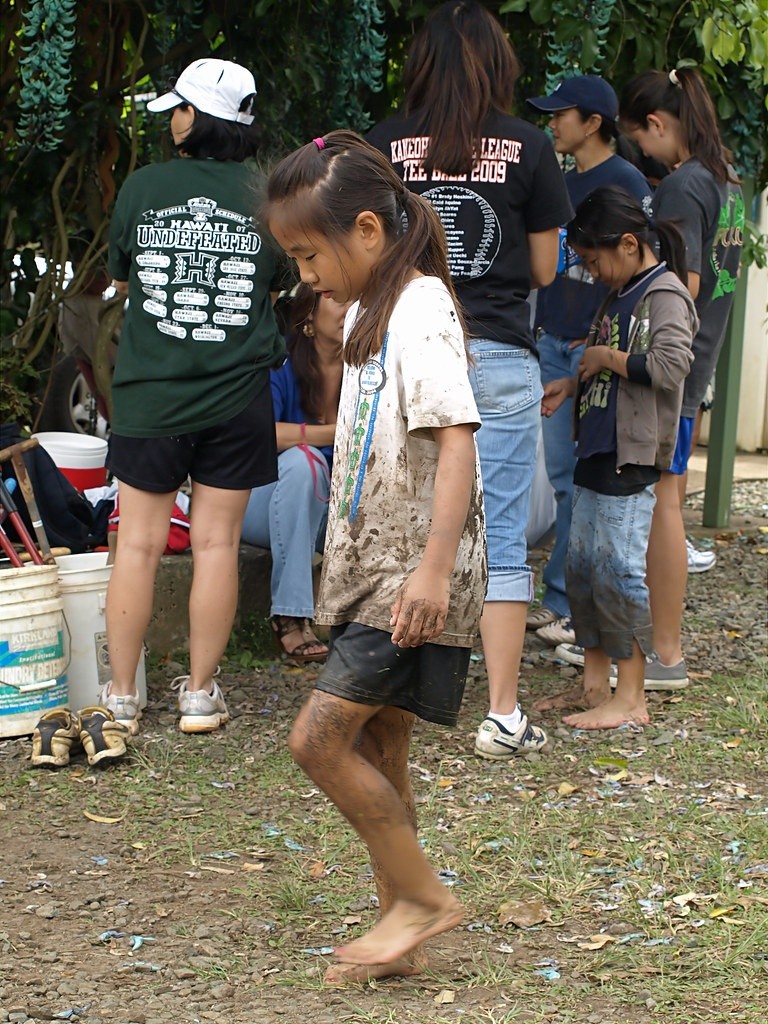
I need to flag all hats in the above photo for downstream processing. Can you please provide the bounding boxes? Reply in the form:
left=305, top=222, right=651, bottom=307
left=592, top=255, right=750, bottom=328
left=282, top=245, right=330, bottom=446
left=525, top=75, right=619, bottom=123
left=147, top=58, right=257, bottom=125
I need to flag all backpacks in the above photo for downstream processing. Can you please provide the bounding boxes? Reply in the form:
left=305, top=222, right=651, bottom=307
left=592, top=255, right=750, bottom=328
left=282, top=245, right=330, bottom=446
left=0, top=423, right=115, bottom=554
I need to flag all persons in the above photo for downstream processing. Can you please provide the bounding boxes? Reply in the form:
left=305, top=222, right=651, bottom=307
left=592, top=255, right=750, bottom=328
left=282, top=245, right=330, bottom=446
left=97, top=58, right=299, bottom=737
left=360, top=0, right=747, bottom=762
left=251, top=129, right=484, bottom=984
left=242, top=277, right=349, bottom=662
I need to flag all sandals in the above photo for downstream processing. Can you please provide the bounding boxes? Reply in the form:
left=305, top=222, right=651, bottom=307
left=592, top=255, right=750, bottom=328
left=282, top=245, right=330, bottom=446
left=269, top=614, right=329, bottom=662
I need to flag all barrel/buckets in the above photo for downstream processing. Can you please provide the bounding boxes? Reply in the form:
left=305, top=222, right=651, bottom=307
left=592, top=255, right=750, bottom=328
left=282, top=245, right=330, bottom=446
left=30, top=431, right=109, bottom=493
left=22, top=551, right=148, bottom=711
left=0, top=565, right=72, bottom=739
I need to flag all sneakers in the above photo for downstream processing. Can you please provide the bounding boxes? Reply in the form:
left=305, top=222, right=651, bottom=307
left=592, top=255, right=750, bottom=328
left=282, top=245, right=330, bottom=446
left=97, top=680, right=142, bottom=736
left=31, top=707, right=132, bottom=772
left=555, top=643, right=689, bottom=690
left=170, top=665, right=229, bottom=732
left=526, top=607, right=576, bottom=647
left=473, top=715, right=547, bottom=761
left=687, top=539, right=716, bottom=573
